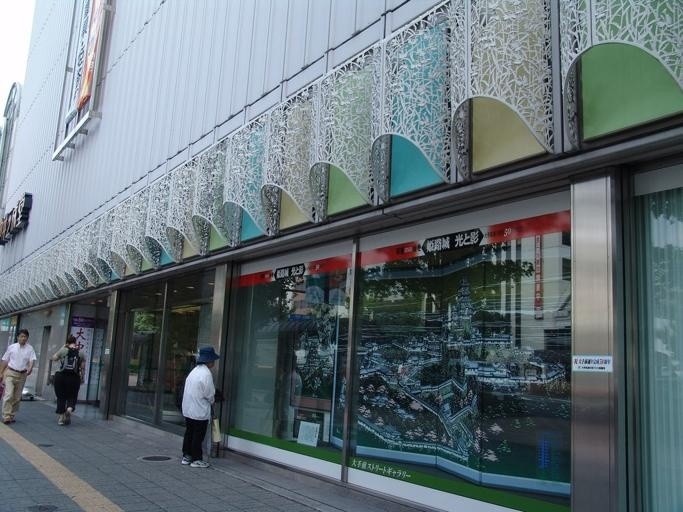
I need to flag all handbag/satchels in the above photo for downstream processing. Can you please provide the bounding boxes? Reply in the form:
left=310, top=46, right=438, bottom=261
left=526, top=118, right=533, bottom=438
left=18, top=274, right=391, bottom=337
left=79, top=369, right=85, bottom=384
left=211, top=419, right=222, bottom=442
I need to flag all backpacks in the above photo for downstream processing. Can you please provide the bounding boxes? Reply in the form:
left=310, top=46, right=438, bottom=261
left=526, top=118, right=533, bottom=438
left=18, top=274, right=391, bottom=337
left=60, top=346, right=80, bottom=376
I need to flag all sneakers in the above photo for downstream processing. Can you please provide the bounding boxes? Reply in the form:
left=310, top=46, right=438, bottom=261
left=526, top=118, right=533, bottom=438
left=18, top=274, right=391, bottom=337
left=3, top=414, right=16, bottom=424
left=56, top=410, right=74, bottom=426
left=181, top=456, right=210, bottom=468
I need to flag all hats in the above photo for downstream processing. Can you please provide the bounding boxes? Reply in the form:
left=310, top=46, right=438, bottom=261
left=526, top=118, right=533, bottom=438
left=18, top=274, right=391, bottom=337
left=197, top=344, right=220, bottom=362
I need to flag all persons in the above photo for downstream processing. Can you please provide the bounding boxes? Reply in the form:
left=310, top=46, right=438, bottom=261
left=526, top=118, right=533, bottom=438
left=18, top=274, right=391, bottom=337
left=52, top=334, right=86, bottom=425
left=0, top=329, right=36, bottom=424
left=181, top=347, right=220, bottom=468
left=280, top=353, right=302, bottom=440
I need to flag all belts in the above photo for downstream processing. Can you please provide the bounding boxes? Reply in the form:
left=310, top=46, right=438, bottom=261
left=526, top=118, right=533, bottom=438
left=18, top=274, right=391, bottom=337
left=8, top=366, right=27, bottom=373
